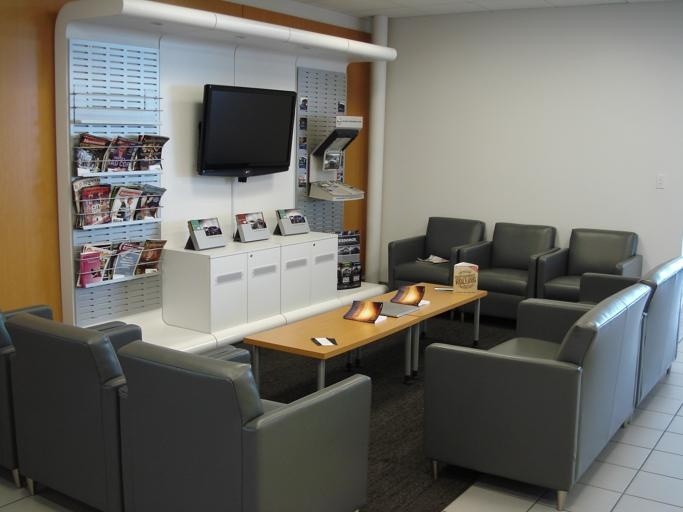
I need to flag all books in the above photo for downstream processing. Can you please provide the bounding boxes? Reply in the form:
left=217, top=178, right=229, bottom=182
left=76, top=238, right=168, bottom=287
left=416, top=254, right=450, bottom=264
left=275, top=208, right=311, bottom=237
left=391, top=285, right=431, bottom=307
left=75, top=132, right=170, bottom=175
left=234, top=210, right=272, bottom=243
left=329, top=229, right=362, bottom=290
left=342, top=300, right=387, bottom=325
left=71, top=177, right=168, bottom=228
left=453, top=261, right=479, bottom=293
left=187, top=217, right=226, bottom=251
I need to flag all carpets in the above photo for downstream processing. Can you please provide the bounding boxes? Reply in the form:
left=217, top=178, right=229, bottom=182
left=219, top=320, right=516, bottom=510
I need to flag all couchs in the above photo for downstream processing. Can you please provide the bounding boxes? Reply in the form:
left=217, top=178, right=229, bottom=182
left=538, top=230, right=643, bottom=296
left=116, top=339, right=373, bottom=512
left=518, top=254, right=683, bottom=430
left=458, top=222, right=562, bottom=325
left=387, top=218, right=485, bottom=322
left=4, top=312, right=235, bottom=511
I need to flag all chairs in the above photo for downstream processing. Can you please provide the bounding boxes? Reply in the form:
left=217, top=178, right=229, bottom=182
left=1, top=306, right=127, bottom=512
left=423, top=283, right=651, bottom=512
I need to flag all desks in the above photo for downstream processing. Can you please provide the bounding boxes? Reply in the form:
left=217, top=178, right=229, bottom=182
left=244, top=303, right=418, bottom=394
left=361, top=281, right=489, bottom=381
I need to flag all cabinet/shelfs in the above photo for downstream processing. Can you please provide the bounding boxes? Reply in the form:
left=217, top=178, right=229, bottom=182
left=282, top=233, right=339, bottom=313
left=54, top=0, right=164, bottom=356
left=161, top=246, right=281, bottom=333
left=297, top=36, right=396, bottom=299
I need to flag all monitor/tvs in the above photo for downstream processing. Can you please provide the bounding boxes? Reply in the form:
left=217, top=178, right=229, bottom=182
left=198, top=84, right=299, bottom=184
